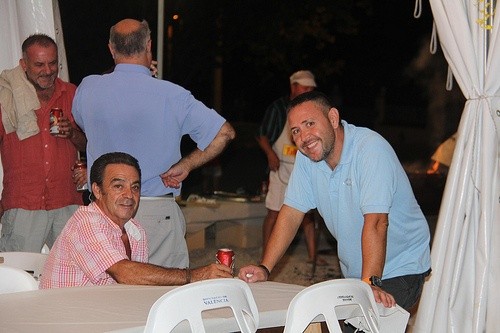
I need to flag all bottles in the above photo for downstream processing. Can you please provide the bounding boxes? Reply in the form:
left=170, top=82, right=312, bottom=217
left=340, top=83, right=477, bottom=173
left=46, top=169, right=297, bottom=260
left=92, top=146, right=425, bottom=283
left=260, top=180, right=267, bottom=199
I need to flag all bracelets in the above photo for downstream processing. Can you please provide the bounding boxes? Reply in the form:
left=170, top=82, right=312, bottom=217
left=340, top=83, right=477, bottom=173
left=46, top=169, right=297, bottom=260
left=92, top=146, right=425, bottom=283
left=257, top=265, right=271, bottom=277
left=181, top=266, right=191, bottom=285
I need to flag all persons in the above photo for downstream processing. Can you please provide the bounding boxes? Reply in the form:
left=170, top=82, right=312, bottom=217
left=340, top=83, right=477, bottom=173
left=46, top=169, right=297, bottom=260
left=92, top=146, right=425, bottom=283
left=253, top=69, right=330, bottom=267
left=230, top=91, right=435, bottom=333
left=71, top=21, right=239, bottom=270
left=71, top=166, right=90, bottom=189
left=0, top=33, right=88, bottom=256
left=40, top=152, right=235, bottom=288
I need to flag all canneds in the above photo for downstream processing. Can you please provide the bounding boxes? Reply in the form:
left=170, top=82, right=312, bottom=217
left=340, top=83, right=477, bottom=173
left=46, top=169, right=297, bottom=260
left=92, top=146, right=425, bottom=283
left=50, top=108, right=64, bottom=135
left=73, top=162, right=89, bottom=190
left=215, top=248, right=235, bottom=278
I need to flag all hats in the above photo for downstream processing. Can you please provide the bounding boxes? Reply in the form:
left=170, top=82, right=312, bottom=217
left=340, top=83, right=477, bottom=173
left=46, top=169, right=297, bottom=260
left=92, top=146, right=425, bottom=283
left=290, top=70, right=317, bottom=87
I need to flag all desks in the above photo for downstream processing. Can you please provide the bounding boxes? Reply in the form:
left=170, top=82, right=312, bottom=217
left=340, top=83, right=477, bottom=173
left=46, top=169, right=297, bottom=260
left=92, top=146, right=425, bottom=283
left=184, top=198, right=267, bottom=249
left=0, top=281, right=397, bottom=333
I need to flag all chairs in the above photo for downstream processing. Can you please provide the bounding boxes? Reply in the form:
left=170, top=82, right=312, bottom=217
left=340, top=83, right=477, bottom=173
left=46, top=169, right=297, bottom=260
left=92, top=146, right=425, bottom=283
left=144, top=278, right=259, bottom=333
left=283, top=278, right=379, bottom=333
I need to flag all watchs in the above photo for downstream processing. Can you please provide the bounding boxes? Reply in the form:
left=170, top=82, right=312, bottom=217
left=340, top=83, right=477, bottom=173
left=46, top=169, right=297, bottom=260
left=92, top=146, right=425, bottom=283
left=360, top=275, right=384, bottom=289
